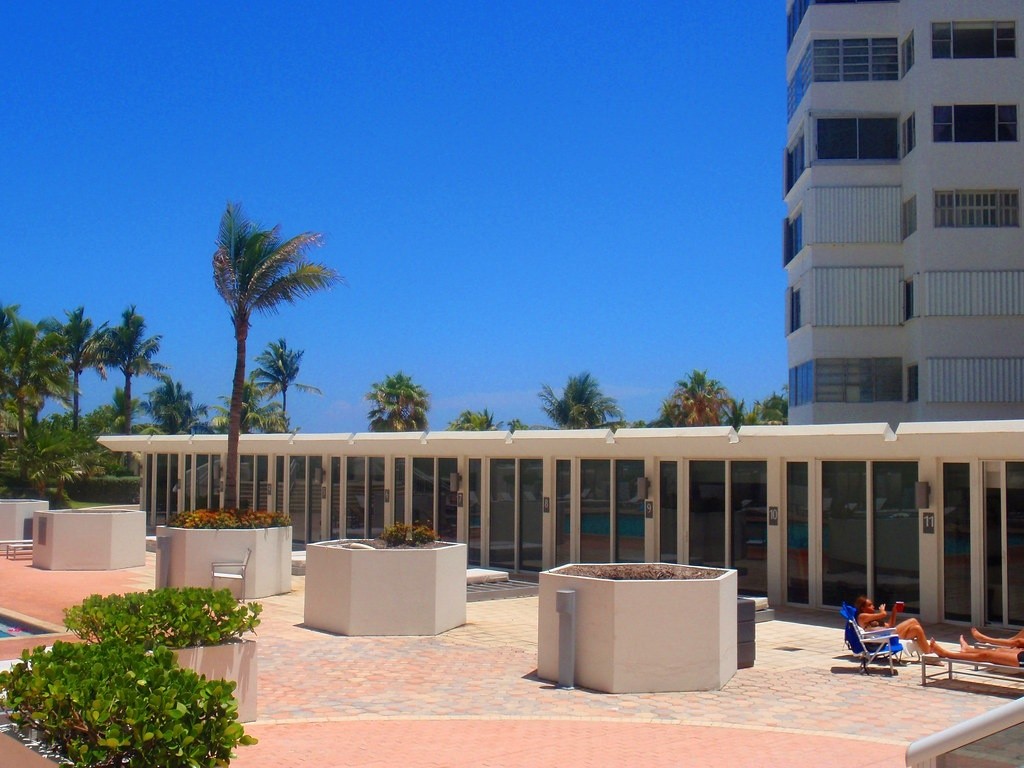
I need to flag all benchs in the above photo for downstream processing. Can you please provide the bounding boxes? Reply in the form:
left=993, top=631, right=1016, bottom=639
left=0, top=539, right=33, bottom=560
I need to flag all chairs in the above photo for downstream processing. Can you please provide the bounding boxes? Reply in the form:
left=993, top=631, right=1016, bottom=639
left=840, top=603, right=1024, bottom=691
left=211, top=548, right=252, bottom=603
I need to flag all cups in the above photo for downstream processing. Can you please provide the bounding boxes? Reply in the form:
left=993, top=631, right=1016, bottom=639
left=896, top=602, right=904, bottom=612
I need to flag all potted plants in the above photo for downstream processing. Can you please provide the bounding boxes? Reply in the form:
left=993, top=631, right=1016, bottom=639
left=63, top=586, right=258, bottom=727
left=303, top=521, right=468, bottom=637
left=156, top=507, right=292, bottom=600
left=537, top=560, right=739, bottom=695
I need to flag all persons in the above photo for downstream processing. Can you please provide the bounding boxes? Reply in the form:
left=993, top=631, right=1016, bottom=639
left=854, top=596, right=1024, bottom=667
